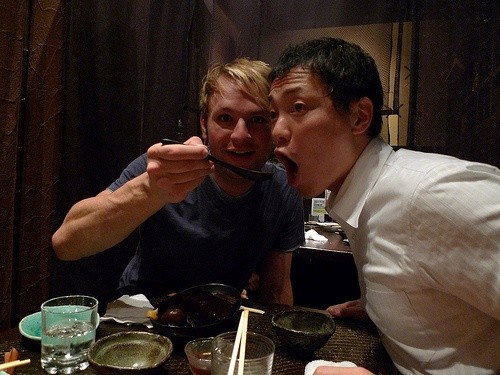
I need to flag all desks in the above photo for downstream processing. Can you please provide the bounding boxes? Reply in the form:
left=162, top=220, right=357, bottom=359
left=291, top=231, right=361, bottom=310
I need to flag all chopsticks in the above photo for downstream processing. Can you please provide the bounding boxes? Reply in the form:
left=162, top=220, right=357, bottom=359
left=227, top=309, right=249, bottom=375
left=0, top=358, right=31, bottom=372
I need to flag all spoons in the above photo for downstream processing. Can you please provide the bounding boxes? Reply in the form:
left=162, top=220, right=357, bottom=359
left=161, top=138, right=275, bottom=181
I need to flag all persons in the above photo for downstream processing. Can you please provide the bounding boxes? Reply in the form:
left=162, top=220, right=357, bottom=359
left=52, top=58, right=306, bottom=307
left=269, top=38, right=500, bottom=375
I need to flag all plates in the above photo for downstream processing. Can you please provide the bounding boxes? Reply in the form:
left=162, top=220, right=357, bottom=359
left=18, top=304, right=100, bottom=347
left=316, top=222, right=339, bottom=231
left=87, top=331, right=174, bottom=375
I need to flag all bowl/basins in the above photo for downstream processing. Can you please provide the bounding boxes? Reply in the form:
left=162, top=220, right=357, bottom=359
left=338, top=230, right=347, bottom=238
left=151, top=282, right=240, bottom=349
left=272, top=310, right=336, bottom=353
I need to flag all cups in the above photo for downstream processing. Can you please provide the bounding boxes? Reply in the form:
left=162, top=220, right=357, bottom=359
left=211, top=331, right=275, bottom=375
left=184, top=337, right=234, bottom=375
left=40, top=295, right=99, bottom=375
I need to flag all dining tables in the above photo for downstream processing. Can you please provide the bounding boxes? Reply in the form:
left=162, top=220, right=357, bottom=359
left=0, top=299, right=398, bottom=375
